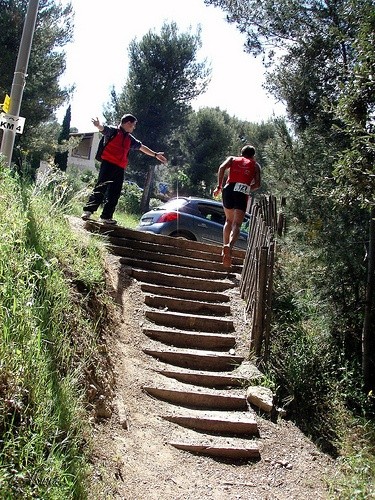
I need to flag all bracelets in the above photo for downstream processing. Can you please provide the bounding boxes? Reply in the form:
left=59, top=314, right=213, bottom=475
left=216, top=185, right=223, bottom=189
left=155, top=152, right=158, bottom=158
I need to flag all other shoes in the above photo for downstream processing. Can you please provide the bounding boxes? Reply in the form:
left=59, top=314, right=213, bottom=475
left=223, top=245, right=232, bottom=269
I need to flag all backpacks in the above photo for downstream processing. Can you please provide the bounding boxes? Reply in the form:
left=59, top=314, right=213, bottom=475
left=95, top=129, right=118, bottom=162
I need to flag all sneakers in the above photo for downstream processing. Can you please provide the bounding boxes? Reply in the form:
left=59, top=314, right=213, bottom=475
left=81, top=211, right=91, bottom=220
left=99, top=218, right=117, bottom=224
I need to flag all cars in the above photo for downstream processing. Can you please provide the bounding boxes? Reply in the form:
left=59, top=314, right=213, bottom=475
left=135, top=196, right=251, bottom=250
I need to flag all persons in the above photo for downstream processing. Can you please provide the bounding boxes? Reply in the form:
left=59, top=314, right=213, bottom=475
left=213, top=144, right=261, bottom=268
left=80, top=113, right=169, bottom=224
